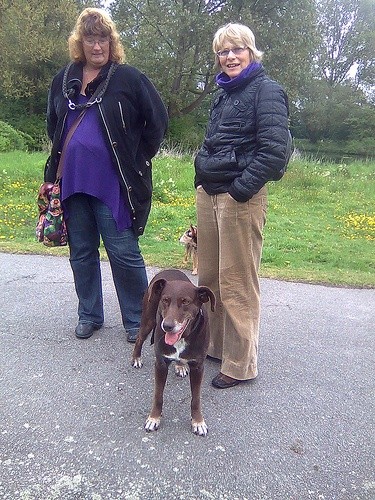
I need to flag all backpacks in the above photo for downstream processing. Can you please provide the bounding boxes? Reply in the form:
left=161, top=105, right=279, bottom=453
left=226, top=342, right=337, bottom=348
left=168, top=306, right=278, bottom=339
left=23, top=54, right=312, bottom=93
left=255, top=79, right=294, bottom=182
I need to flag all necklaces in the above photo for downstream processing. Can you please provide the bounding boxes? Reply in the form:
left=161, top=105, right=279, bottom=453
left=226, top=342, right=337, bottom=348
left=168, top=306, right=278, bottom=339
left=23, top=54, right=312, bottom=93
left=83, top=66, right=89, bottom=82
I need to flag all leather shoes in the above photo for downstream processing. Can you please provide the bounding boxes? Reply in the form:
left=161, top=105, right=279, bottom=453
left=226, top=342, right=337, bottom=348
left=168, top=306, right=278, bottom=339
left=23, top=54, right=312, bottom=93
left=212, top=372, right=240, bottom=388
left=128, top=330, right=139, bottom=342
left=75, top=322, right=102, bottom=338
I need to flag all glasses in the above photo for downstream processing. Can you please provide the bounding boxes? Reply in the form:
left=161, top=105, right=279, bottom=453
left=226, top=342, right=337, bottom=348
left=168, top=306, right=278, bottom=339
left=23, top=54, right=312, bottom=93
left=83, top=37, right=109, bottom=45
left=217, top=47, right=248, bottom=55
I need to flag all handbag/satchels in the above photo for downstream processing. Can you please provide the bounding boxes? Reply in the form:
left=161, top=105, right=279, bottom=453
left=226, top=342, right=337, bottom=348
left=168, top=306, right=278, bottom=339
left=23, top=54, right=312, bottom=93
left=36, top=182, right=69, bottom=247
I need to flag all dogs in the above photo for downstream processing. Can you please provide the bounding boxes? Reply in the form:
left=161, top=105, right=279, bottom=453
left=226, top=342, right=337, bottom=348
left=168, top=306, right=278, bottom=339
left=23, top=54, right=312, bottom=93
left=131, top=266, right=218, bottom=437
left=178, top=223, right=198, bottom=276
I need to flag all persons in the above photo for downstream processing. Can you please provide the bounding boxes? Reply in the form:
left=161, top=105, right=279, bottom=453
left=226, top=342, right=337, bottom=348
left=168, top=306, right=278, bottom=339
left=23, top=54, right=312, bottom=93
left=44, top=8, right=168, bottom=343
left=193, top=22, right=290, bottom=389
